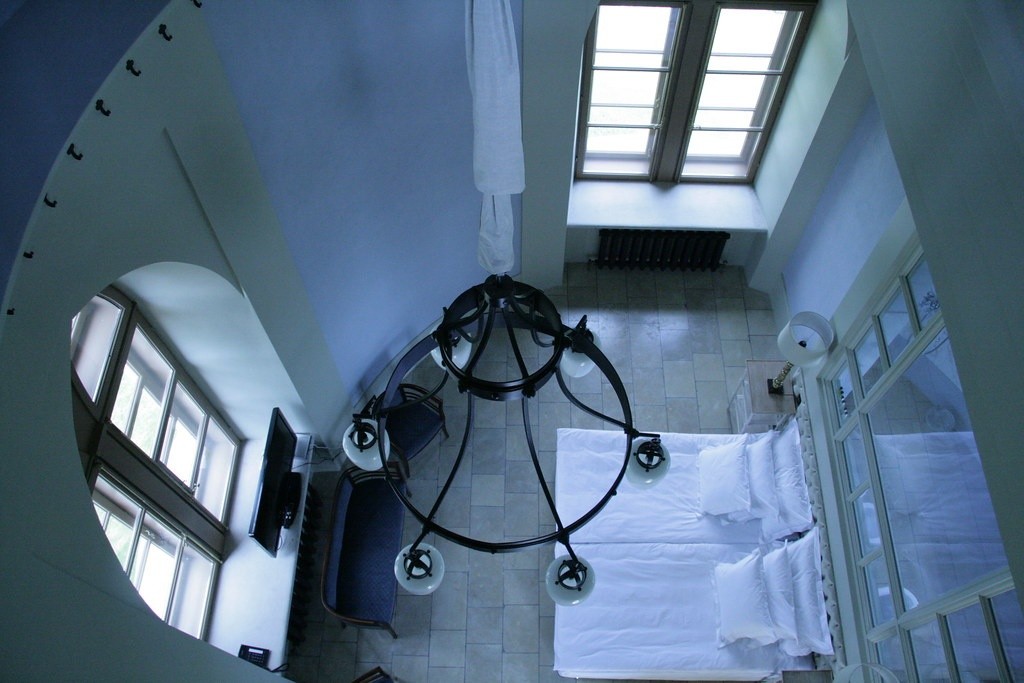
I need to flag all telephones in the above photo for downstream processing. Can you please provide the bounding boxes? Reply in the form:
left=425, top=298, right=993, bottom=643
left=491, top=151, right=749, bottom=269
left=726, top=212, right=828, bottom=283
left=238, top=644, right=269, bottom=668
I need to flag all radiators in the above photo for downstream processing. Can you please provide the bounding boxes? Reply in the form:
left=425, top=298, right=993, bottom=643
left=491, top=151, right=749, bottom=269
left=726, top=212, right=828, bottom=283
left=586, top=229, right=730, bottom=273
left=287, top=483, right=322, bottom=645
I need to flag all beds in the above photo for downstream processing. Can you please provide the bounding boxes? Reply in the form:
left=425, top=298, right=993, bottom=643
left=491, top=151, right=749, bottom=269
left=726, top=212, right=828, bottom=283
left=840, top=430, right=1024, bottom=683
left=551, top=404, right=847, bottom=681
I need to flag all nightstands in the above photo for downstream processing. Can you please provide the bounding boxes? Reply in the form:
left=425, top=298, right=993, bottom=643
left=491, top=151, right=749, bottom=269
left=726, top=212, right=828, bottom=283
left=725, top=360, right=797, bottom=434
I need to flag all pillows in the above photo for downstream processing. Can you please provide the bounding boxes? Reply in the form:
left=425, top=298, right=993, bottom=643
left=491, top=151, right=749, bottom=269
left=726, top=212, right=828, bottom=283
left=707, top=547, right=778, bottom=651
left=780, top=527, right=835, bottom=656
left=720, top=429, right=780, bottom=526
left=742, top=540, right=800, bottom=649
left=696, top=433, right=751, bottom=517
left=759, top=417, right=815, bottom=545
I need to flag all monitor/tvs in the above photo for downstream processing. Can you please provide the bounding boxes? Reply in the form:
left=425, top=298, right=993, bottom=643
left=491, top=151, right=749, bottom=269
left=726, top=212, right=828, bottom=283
left=248, top=408, right=303, bottom=559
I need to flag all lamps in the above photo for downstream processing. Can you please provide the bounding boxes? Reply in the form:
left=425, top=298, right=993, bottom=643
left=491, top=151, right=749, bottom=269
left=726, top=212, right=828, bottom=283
left=767, top=312, right=834, bottom=395
left=341, top=1, right=670, bottom=609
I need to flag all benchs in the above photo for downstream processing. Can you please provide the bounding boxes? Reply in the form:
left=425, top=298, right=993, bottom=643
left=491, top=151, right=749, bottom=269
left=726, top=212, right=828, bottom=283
left=320, top=461, right=413, bottom=640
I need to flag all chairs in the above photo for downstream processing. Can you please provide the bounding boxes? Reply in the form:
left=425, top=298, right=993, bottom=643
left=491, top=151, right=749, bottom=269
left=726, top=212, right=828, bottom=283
left=370, top=383, right=449, bottom=479
